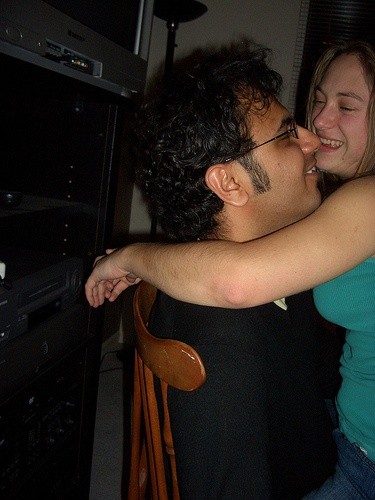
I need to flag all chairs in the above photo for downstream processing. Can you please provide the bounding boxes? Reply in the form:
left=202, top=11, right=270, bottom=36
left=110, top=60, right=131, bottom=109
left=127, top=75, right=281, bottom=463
left=124, top=280, right=206, bottom=500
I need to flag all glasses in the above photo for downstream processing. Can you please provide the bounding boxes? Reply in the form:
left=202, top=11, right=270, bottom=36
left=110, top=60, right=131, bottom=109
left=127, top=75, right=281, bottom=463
left=219, top=121, right=298, bottom=163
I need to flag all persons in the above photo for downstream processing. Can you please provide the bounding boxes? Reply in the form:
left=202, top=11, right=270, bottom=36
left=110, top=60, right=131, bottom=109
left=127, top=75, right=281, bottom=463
left=134, top=42, right=333, bottom=500
left=84, top=39, right=374, bottom=499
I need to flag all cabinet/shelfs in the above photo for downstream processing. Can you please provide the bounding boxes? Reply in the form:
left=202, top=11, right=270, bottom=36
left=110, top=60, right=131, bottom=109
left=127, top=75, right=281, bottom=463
left=1, top=50, right=152, bottom=500
